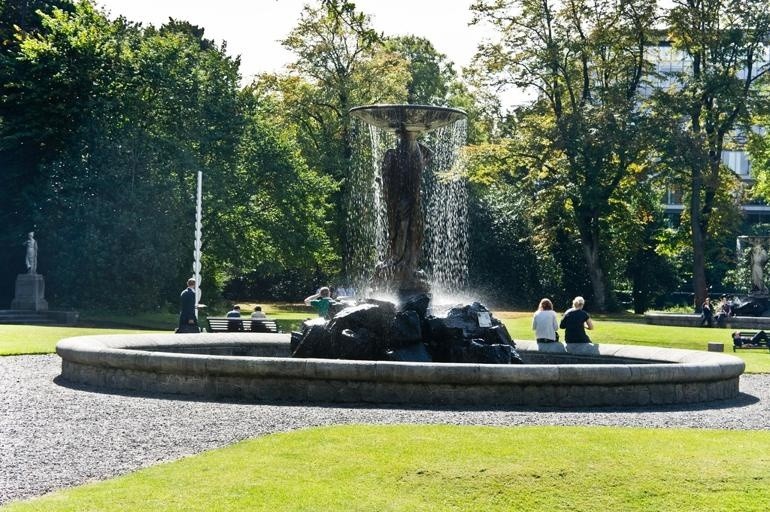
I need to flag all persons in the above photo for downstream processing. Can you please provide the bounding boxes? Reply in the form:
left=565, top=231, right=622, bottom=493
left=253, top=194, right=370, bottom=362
left=227, top=306, right=241, bottom=330
left=316, top=283, right=359, bottom=300
left=749, top=239, right=769, bottom=299
left=250, top=305, right=267, bottom=330
left=713, top=296, right=732, bottom=328
left=22, top=231, right=39, bottom=274
left=732, top=330, right=770, bottom=348
left=701, top=297, right=714, bottom=327
left=532, top=298, right=560, bottom=345
left=175, top=278, right=198, bottom=333
left=303, top=286, right=336, bottom=317
left=559, top=295, right=594, bottom=343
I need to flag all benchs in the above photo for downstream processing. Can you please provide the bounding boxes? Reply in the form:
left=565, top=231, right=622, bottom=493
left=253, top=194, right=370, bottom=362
left=204, top=316, right=278, bottom=333
left=733, top=332, right=770, bottom=351
